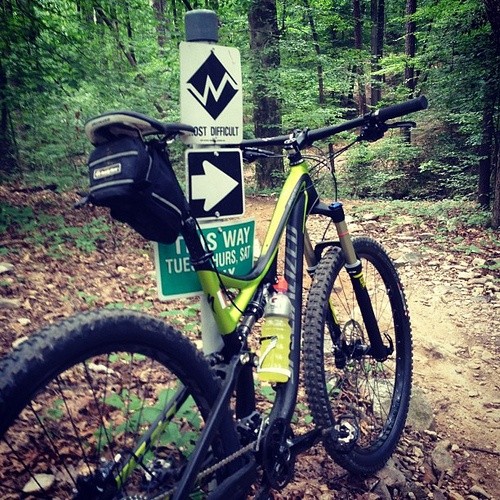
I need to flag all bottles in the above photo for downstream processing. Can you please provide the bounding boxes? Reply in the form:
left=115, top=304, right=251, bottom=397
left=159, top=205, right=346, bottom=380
left=256, top=275, right=294, bottom=382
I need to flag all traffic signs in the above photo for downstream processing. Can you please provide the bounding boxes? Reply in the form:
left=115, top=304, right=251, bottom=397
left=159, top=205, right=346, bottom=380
left=152, top=217, right=255, bottom=303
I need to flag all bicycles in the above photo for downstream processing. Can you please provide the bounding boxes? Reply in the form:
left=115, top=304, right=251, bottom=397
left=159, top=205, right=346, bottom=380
left=0, top=96, right=429, bottom=500
